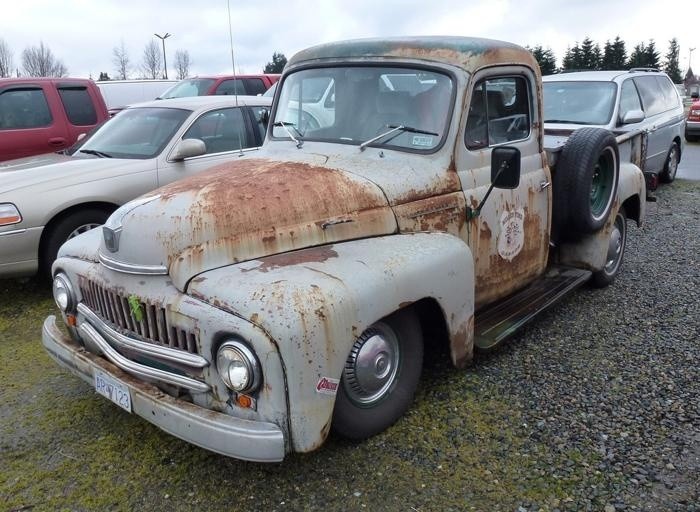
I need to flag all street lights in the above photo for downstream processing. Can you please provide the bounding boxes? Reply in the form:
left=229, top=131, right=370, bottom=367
left=154, top=33, right=172, bottom=80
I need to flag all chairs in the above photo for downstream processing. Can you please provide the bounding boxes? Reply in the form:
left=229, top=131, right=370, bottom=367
left=363, top=90, right=513, bottom=148
left=206, top=123, right=244, bottom=153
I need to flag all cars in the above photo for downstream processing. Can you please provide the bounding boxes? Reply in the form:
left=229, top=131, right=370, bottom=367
left=685, top=91, right=699, bottom=142
left=41, top=36, right=644, bottom=466
left=0, top=95, right=334, bottom=278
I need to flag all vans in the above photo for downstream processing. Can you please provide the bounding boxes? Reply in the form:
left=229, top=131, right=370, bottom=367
left=537, top=67, right=686, bottom=181
